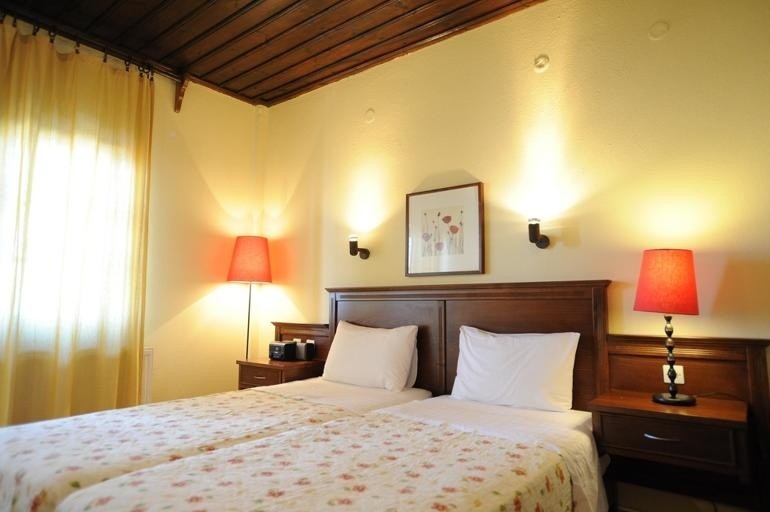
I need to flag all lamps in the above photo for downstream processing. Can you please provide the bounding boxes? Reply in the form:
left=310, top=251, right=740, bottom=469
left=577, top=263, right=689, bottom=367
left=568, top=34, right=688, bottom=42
left=633, top=247, right=701, bottom=407
left=348, top=234, right=371, bottom=260
left=524, top=218, right=550, bottom=250
left=226, top=234, right=274, bottom=359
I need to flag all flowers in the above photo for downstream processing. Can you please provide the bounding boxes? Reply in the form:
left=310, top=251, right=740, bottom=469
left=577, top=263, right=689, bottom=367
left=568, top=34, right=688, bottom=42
left=420, top=209, right=463, bottom=256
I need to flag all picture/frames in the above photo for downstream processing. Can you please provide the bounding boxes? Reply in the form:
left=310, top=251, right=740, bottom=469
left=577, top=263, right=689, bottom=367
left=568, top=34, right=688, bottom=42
left=403, top=180, right=491, bottom=279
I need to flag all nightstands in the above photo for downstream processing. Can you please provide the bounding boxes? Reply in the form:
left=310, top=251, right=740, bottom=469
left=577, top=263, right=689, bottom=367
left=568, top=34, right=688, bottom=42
left=588, top=390, right=755, bottom=512
left=236, top=357, right=320, bottom=394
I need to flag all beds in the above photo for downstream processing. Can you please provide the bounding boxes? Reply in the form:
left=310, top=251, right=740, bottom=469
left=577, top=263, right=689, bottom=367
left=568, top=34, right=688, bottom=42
left=0, top=278, right=615, bottom=512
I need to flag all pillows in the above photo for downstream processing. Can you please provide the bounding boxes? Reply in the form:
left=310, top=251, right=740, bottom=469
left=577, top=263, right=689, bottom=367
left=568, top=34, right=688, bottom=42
left=449, top=323, right=583, bottom=414
left=320, top=319, right=420, bottom=393
